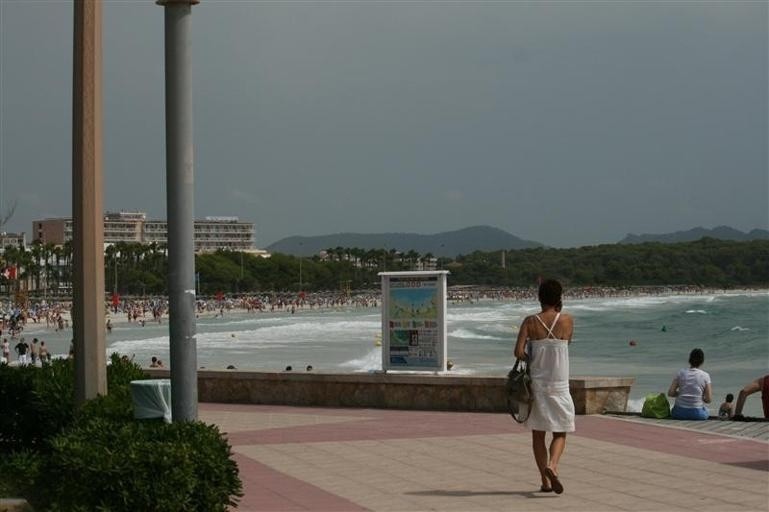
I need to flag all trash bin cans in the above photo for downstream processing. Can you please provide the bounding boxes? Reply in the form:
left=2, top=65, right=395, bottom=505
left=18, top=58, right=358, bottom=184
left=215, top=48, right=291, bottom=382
left=130, top=379, right=173, bottom=424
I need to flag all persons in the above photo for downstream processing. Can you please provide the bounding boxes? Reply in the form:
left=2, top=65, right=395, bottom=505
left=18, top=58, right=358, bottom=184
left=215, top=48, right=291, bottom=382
left=719, top=393, right=735, bottom=417
left=666, top=347, right=714, bottom=420
left=731, top=373, right=769, bottom=421
left=512, top=279, right=576, bottom=494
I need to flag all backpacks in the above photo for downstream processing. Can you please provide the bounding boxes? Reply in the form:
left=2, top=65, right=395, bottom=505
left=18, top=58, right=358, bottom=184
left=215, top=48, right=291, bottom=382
left=640, top=393, right=670, bottom=419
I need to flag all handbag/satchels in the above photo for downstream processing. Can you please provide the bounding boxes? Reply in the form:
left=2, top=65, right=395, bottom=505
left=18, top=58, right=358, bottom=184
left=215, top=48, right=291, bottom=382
left=506, top=352, right=534, bottom=423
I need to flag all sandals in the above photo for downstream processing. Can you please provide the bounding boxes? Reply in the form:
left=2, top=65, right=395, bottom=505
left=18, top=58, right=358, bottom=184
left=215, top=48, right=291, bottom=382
left=541, top=466, right=563, bottom=495
